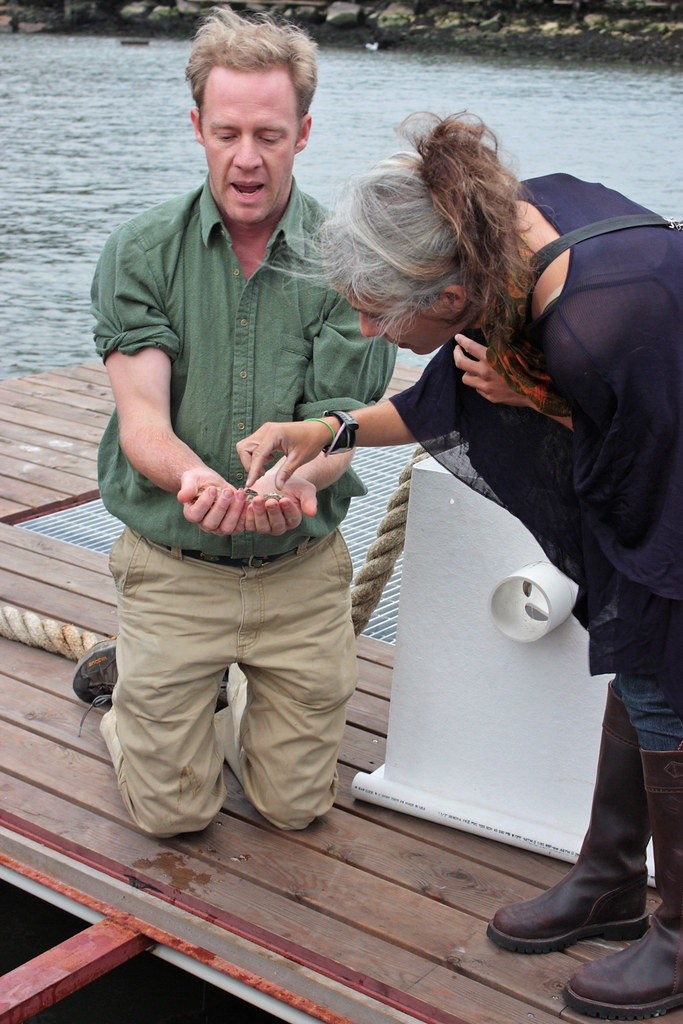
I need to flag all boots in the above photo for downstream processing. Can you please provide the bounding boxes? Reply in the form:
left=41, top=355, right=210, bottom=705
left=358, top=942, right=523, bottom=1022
left=562, top=747, right=683, bottom=1022
left=487, top=680, right=650, bottom=953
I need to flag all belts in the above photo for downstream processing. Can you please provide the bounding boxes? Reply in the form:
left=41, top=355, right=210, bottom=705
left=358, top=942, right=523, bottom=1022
left=167, top=536, right=316, bottom=569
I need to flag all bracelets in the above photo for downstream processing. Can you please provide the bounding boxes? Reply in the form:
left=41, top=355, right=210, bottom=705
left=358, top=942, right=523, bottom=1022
left=304, top=418, right=334, bottom=442
left=322, top=410, right=359, bottom=454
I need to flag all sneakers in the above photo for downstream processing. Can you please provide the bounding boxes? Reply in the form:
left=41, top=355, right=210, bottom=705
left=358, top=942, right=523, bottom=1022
left=72, top=637, right=229, bottom=737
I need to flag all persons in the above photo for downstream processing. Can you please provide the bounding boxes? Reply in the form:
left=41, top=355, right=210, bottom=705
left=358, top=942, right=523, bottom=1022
left=236, top=111, right=683, bottom=1020
left=71, top=8, right=396, bottom=839
